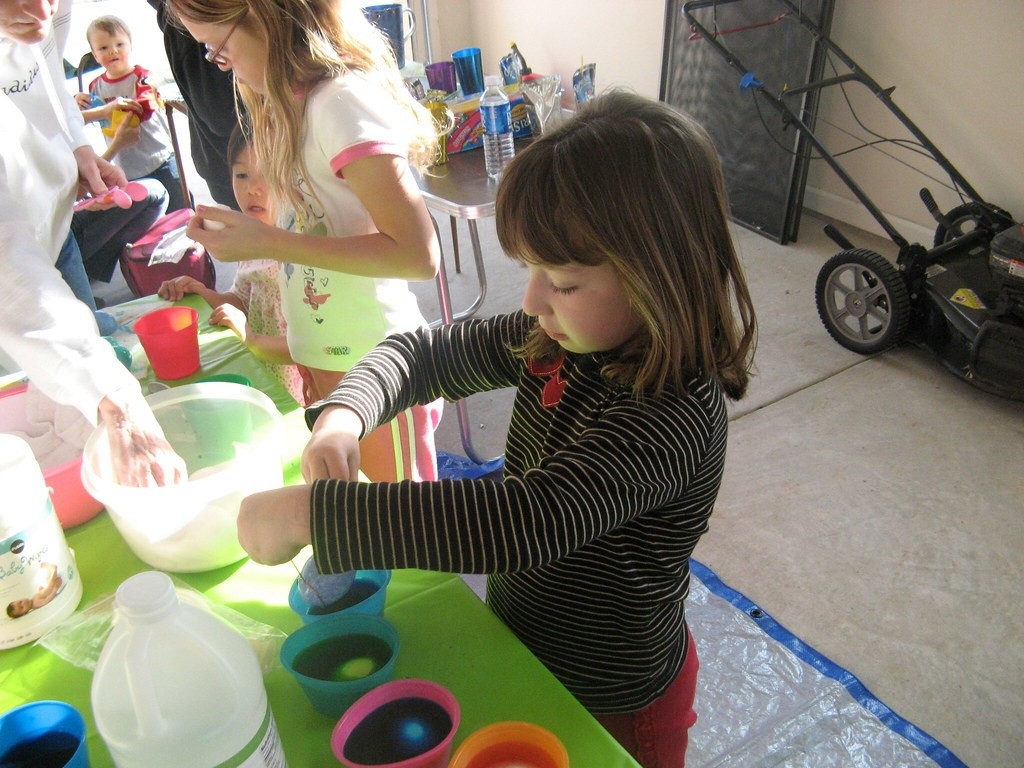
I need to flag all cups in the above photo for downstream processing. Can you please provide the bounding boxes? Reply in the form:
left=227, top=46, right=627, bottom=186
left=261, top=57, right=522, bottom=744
left=1, top=700, right=91, bottom=768
left=187, top=374, right=252, bottom=462
left=452, top=48, right=485, bottom=97
left=426, top=61, right=457, bottom=95
left=134, top=306, right=200, bottom=379
left=362, top=4, right=416, bottom=70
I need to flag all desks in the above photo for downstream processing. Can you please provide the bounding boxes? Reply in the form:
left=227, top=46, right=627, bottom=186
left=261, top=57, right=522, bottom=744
left=0, top=293, right=644, bottom=768
left=149, top=64, right=576, bottom=469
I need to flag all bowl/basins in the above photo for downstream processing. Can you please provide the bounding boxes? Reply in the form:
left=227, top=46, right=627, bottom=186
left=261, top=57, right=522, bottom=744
left=448, top=721, right=568, bottom=768
left=1, top=372, right=104, bottom=530
left=332, top=681, right=460, bottom=768
left=289, top=570, right=392, bottom=623
left=80, top=381, right=285, bottom=573
left=280, top=612, right=401, bottom=717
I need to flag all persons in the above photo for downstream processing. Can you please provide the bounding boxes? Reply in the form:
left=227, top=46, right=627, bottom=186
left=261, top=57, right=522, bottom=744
left=165, top=0, right=446, bottom=483
left=236, top=93, right=759, bottom=768
left=158, top=113, right=307, bottom=411
left=6, top=562, right=62, bottom=619
left=70, top=0, right=244, bottom=309
left=0, top=0, right=188, bottom=488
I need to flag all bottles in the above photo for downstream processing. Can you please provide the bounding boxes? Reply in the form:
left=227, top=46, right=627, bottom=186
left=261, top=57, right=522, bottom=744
left=91, top=570, right=287, bottom=768
left=86, top=93, right=108, bottom=123
left=0, top=436, right=83, bottom=653
left=519, top=68, right=541, bottom=136
left=478, top=76, right=514, bottom=178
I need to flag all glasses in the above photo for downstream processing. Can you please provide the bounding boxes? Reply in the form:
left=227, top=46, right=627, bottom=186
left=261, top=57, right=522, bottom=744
left=206, top=10, right=248, bottom=66
